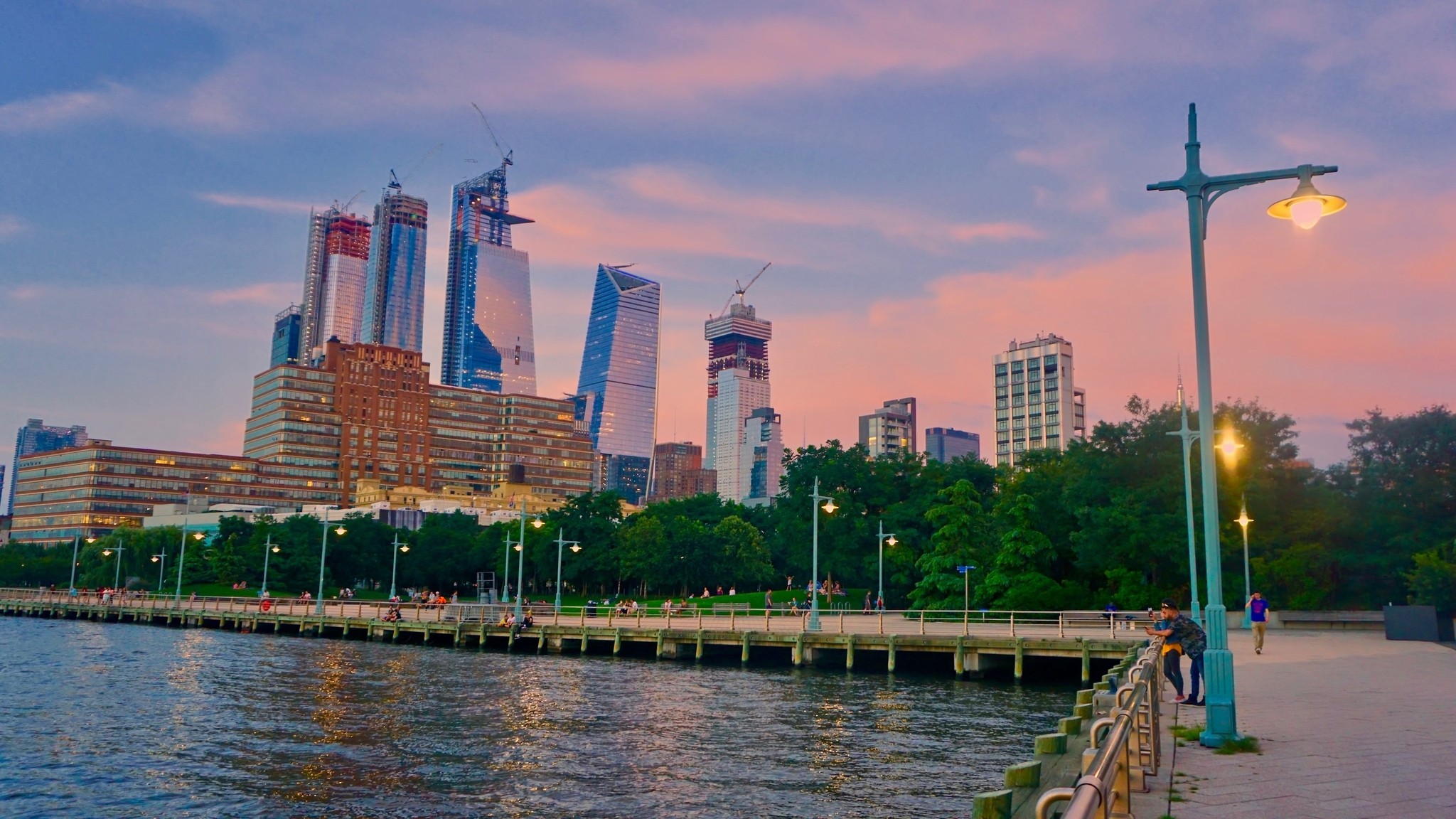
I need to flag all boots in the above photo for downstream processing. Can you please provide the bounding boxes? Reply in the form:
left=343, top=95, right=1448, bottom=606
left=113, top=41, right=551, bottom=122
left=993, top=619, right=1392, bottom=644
left=1193, top=695, right=1206, bottom=708
left=1179, top=694, right=1197, bottom=706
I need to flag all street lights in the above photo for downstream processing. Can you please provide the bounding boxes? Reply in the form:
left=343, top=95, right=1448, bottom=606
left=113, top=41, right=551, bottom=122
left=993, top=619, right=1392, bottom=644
left=313, top=507, right=348, bottom=615
left=1146, top=103, right=1347, bottom=753
left=502, top=530, right=522, bottom=603
left=1166, top=401, right=1246, bottom=626
left=68, top=525, right=96, bottom=597
left=875, top=520, right=898, bottom=614
left=807, top=475, right=839, bottom=632
left=553, top=527, right=582, bottom=613
left=261, top=534, right=281, bottom=597
left=515, top=498, right=545, bottom=623
left=170, top=518, right=205, bottom=611
left=150, top=547, right=168, bottom=591
left=1233, top=502, right=1255, bottom=629
left=388, top=533, right=410, bottom=599
left=102, top=540, right=126, bottom=588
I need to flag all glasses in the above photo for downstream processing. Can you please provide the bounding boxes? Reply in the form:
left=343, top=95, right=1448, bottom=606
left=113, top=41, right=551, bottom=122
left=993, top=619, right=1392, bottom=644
left=1162, top=602, right=1172, bottom=609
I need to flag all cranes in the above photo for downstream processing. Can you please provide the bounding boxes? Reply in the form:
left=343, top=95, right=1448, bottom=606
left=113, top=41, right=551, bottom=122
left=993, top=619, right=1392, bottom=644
left=736, top=262, right=771, bottom=305
left=329, top=190, right=366, bottom=218
left=710, top=294, right=735, bottom=323
left=387, top=142, right=445, bottom=194
left=469, top=101, right=513, bottom=245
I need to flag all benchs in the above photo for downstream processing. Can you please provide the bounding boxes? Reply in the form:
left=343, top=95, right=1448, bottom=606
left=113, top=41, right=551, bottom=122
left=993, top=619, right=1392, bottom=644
left=1278, top=610, right=1384, bottom=628
left=121, top=591, right=150, bottom=600
left=771, top=603, right=792, bottom=615
left=711, top=602, right=751, bottom=618
left=612, top=603, right=648, bottom=618
left=658, top=603, right=697, bottom=618
left=1062, top=610, right=1205, bottom=629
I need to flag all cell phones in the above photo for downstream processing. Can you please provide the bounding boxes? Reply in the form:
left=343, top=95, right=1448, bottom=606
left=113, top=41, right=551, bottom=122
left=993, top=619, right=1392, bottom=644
left=1145, top=626, right=1148, bottom=630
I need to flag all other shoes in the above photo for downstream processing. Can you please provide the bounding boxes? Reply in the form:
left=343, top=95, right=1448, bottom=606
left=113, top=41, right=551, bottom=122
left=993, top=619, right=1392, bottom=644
left=1260, top=651, right=1262, bottom=654
left=1256, top=648, right=1261, bottom=654
left=1169, top=698, right=1186, bottom=704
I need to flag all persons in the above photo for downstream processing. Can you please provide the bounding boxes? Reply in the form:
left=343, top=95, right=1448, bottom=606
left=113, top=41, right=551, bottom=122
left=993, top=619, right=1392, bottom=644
left=701, top=587, right=709, bottom=598
left=765, top=589, right=772, bottom=619
left=513, top=592, right=547, bottom=615
left=808, top=580, right=840, bottom=594
left=788, top=592, right=813, bottom=620
left=190, top=590, right=198, bottom=601
left=717, top=587, right=723, bottom=595
left=878, top=596, right=883, bottom=615
left=664, top=598, right=687, bottom=618
left=50, top=584, right=56, bottom=594
left=261, top=590, right=270, bottom=600
left=339, top=586, right=357, bottom=600
left=1102, top=601, right=1117, bottom=628
left=1145, top=599, right=1208, bottom=707
left=1246, top=590, right=1269, bottom=654
left=298, top=591, right=311, bottom=605
left=382, top=589, right=459, bottom=622
left=498, top=613, right=533, bottom=639
left=863, top=591, right=873, bottom=615
left=233, top=581, right=249, bottom=589
left=689, top=593, right=694, bottom=598
left=784, top=575, right=794, bottom=591
left=615, top=598, right=638, bottom=619
left=71, top=585, right=146, bottom=606
left=729, top=587, right=735, bottom=595
